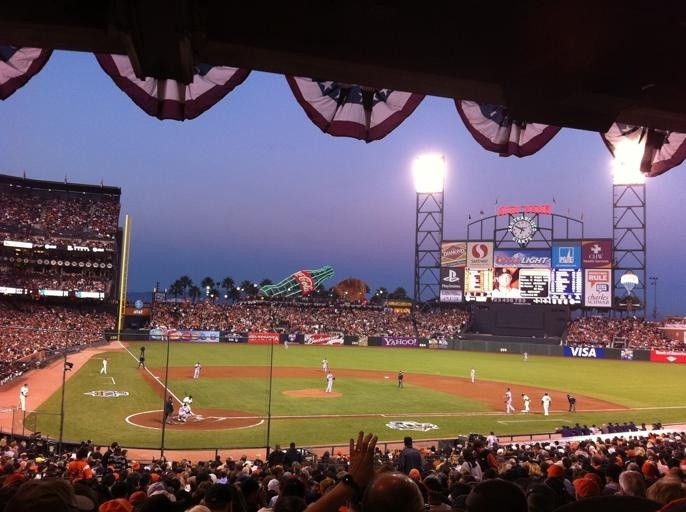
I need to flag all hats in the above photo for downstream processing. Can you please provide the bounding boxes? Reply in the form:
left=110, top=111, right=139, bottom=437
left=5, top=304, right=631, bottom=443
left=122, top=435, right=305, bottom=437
left=497, top=269, right=510, bottom=276
left=544, top=446, right=564, bottom=454
left=572, top=478, right=598, bottom=497
left=607, top=447, right=635, bottom=467
left=129, top=463, right=185, bottom=502
left=409, top=469, right=420, bottom=478
left=547, top=465, right=564, bottom=477
left=0, top=477, right=94, bottom=512
left=469, top=440, right=483, bottom=451
left=99, top=498, right=134, bottom=512
left=102, top=472, right=120, bottom=484
left=497, top=449, right=504, bottom=455
left=642, top=460, right=655, bottom=476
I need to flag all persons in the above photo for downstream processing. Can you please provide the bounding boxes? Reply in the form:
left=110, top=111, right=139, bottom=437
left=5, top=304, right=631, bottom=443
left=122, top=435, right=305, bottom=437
left=1, top=186, right=120, bottom=293
left=0, top=298, right=685, bottom=511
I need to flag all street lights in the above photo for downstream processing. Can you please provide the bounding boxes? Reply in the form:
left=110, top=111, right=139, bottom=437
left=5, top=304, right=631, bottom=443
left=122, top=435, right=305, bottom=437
left=649, top=276, right=659, bottom=320
